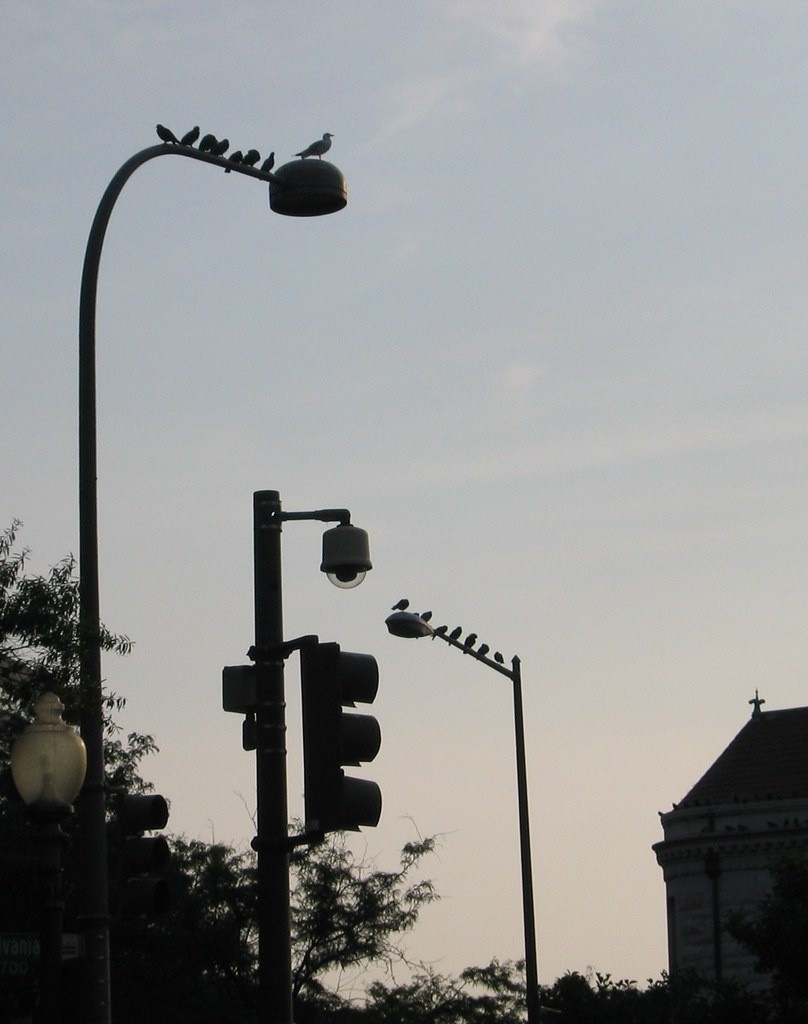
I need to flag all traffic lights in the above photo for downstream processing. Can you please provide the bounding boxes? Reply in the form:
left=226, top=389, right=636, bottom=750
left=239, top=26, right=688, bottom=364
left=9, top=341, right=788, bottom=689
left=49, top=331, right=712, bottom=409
left=221, top=665, right=257, bottom=713
left=104, top=788, right=171, bottom=920
left=299, top=640, right=383, bottom=835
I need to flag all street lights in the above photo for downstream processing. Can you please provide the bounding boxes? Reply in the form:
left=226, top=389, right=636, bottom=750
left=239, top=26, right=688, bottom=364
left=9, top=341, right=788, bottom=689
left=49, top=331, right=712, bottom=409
left=384, top=613, right=542, bottom=1024
left=78, top=142, right=350, bottom=1024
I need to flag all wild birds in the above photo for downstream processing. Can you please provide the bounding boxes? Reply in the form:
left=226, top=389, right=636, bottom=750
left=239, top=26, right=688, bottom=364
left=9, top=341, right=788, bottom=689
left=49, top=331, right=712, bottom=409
left=181, top=126, right=200, bottom=147
left=261, top=151, right=275, bottom=172
left=476, top=643, right=489, bottom=662
left=658, top=790, right=808, bottom=834
left=494, top=651, right=505, bottom=664
left=291, top=132, right=335, bottom=161
left=198, top=134, right=230, bottom=157
left=463, top=632, right=478, bottom=654
left=155, top=124, right=181, bottom=146
left=241, top=149, right=261, bottom=168
left=432, top=625, right=448, bottom=641
left=449, top=625, right=463, bottom=647
left=413, top=610, right=433, bottom=623
left=224, top=151, right=243, bottom=174
left=391, top=599, right=409, bottom=612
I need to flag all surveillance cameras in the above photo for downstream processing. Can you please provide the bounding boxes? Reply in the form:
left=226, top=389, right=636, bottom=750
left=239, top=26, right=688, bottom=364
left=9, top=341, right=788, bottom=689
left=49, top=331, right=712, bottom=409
left=320, top=525, right=373, bottom=590
